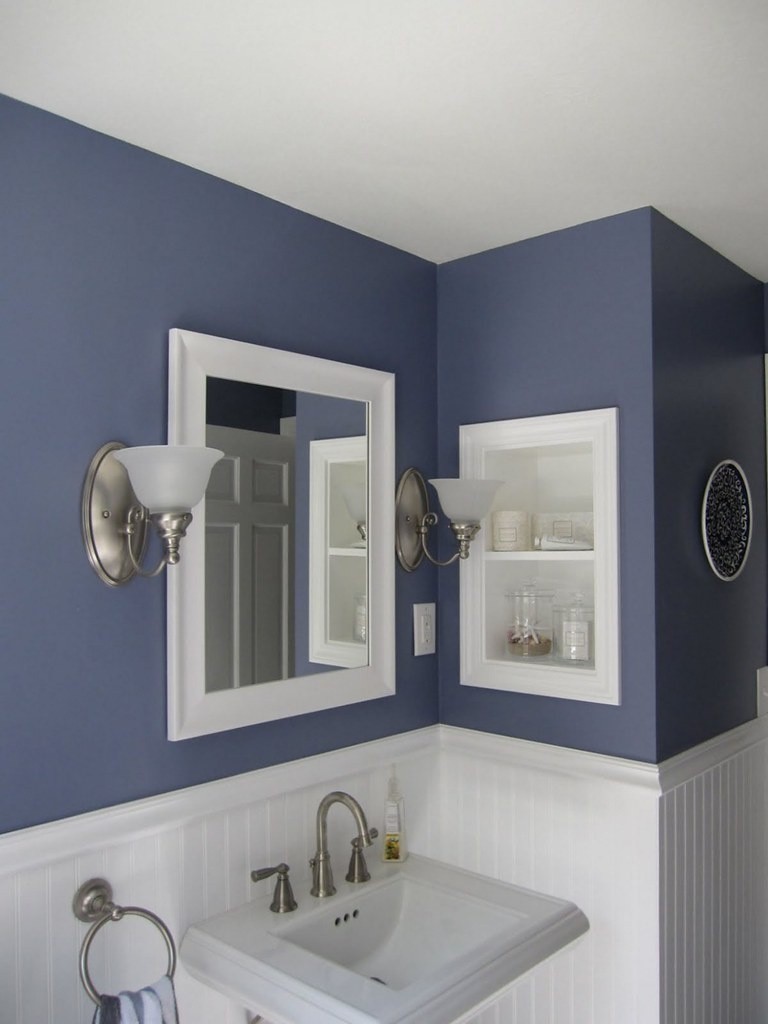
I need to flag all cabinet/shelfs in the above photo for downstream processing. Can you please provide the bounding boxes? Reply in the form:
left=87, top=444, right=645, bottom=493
left=460, top=406, right=624, bottom=706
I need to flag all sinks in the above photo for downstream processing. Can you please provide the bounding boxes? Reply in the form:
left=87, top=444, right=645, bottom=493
left=179, top=838, right=591, bottom=1023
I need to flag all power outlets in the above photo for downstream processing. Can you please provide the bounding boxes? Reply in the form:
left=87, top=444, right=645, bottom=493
left=412, top=602, right=436, bottom=657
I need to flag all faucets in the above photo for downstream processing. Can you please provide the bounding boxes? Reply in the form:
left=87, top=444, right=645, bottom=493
left=309, top=791, right=375, bottom=898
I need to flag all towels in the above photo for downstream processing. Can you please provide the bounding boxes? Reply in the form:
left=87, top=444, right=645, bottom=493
left=90, top=975, right=179, bottom=1024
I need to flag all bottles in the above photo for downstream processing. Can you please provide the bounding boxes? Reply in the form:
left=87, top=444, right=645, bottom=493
left=382, top=777, right=409, bottom=864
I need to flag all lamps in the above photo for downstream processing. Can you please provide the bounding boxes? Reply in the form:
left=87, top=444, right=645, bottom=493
left=79, top=440, right=227, bottom=589
left=397, top=467, right=504, bottom=573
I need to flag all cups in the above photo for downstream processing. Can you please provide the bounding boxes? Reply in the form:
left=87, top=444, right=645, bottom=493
left=493, top=510, right=528, bottom=551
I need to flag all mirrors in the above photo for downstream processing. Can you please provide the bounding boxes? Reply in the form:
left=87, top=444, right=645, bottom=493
left=165, top=327, right=397, bottom=742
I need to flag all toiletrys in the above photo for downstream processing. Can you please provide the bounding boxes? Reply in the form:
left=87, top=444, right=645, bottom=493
left=380, top=763, right=407, bottom=862
